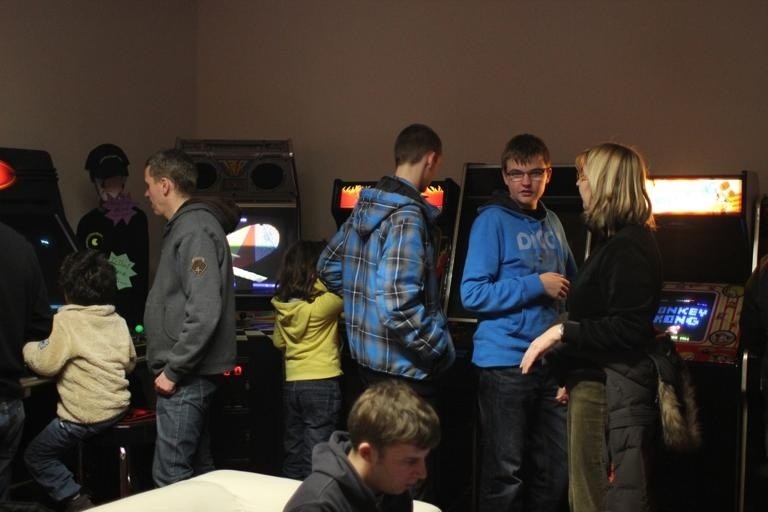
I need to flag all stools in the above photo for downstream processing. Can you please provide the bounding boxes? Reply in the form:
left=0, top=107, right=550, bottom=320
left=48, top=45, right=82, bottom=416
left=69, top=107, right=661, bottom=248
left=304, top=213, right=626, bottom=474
left=112, top=408, right=157, bottom=498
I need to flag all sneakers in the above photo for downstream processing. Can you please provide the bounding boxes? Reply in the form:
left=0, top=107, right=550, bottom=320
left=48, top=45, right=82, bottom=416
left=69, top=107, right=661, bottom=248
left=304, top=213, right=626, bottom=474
left=67, top=495, right=94, bottom=512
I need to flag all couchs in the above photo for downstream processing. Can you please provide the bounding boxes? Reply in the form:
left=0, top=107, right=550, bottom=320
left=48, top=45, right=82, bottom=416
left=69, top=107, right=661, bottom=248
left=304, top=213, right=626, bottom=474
left=78, top=469, right=440, bottom=512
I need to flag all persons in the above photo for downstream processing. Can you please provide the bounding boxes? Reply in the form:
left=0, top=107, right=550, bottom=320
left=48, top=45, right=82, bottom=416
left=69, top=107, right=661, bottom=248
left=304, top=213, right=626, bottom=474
left=21, top=247, right=137, bottom=511
left=316, top=126, right=456, bottom=512
left=0, top=162, right=53, bottom=506
left=285, top=378, right=442, bottom=512
left=142, top=149, right=237, bottom=488
left=76, top=143, right=150, bottom=274
left=739, top=254, right=767, bottom=359
left=518, top=143, right=661, bottom=512
left=459, top=133, right=578, bottom=512
left=270, top=240, right=343, bottom=478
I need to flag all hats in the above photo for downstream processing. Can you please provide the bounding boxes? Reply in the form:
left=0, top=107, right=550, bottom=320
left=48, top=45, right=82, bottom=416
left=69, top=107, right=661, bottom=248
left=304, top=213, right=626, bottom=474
left=85, top=144, right=131, bottom=179
left=0, top=160, right=19, bottom=188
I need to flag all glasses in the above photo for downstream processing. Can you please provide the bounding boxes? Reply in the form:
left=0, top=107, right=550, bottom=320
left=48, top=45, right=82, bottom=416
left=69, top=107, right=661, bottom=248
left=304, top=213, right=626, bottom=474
left=508, top=168, right=547, bottom=182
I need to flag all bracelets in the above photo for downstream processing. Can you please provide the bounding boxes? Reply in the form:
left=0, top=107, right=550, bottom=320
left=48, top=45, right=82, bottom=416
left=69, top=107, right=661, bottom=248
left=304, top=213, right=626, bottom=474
left=558, top=323, right=565, bottom=338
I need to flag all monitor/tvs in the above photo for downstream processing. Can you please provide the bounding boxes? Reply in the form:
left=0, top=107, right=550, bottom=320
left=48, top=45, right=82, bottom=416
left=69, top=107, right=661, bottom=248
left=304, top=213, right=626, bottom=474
left=650, top=285, right=719, bottom=344
left=220, top=209, right=289, bottom=299
left=10, top=216, right=78, bottom=309
